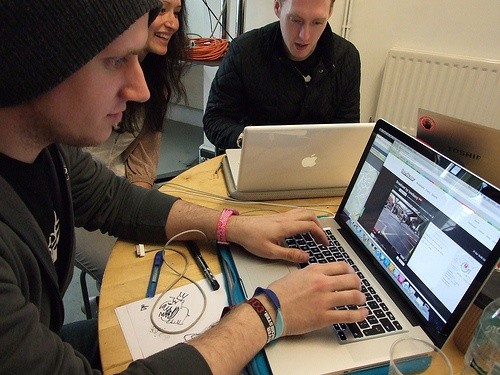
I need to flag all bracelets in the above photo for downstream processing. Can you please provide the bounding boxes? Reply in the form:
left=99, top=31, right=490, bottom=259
left=217, top=208, right=239, bottom=245
left=247, top=287, right=284, bottom=343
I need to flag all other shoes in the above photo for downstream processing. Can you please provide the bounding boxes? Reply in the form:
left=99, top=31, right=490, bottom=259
left=81, top=296, right=98, bottom=318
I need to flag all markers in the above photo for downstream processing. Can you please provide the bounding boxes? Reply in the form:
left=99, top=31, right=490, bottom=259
left=185, top=240, right=220, bottom=290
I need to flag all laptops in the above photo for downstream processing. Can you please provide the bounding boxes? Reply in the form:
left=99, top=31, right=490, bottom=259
left=226, top=108, right=500, bottom=375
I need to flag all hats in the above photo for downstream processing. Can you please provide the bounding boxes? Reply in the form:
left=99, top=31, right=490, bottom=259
left=0, top=0, right=162, bottom=107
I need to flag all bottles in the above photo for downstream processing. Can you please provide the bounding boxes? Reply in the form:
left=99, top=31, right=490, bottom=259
left=459, top=296, right=500, bottom=374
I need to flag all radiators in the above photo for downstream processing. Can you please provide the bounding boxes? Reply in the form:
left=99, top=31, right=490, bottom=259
left=373, top=48, right=500, bottom=138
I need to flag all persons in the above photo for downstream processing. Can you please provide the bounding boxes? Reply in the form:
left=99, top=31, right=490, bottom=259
left=0, top=0, right=369, bottom=375
left=74, top=0, right=193, bottom=318
left=202, top=0, right=361, bottom=157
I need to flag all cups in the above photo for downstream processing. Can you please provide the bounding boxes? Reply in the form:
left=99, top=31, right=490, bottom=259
left=387, top=336, right=454, bottom=375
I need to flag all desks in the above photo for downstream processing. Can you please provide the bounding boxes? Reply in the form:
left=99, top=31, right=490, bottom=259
left=99, top=150, right=475, bottom=375
